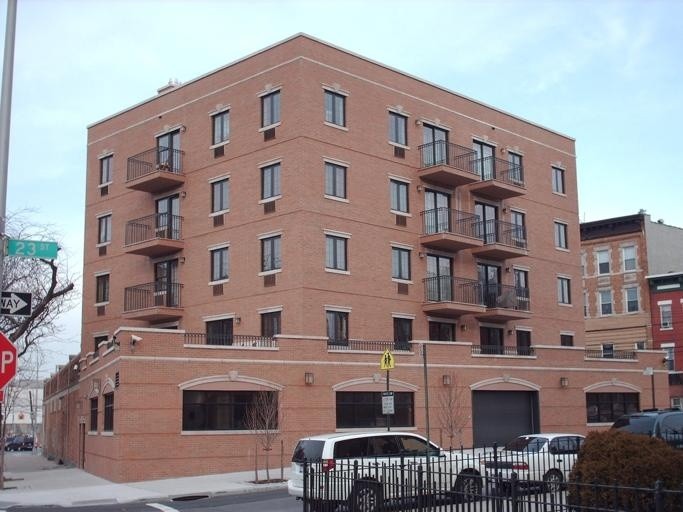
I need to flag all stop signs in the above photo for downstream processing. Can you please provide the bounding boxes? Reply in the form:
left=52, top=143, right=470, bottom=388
left=0, top=331, right=17, bottom=392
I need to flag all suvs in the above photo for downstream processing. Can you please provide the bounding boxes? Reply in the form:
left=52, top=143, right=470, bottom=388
left=287, top=429, right=487, bottom=512
left=609, top=407, right=682, bottom=451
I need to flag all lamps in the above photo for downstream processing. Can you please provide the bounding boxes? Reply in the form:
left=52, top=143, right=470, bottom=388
left=442, top=374, right=450, bottom=386
left=561, top=377, right=568, bottom=388
left=303, top=371, right=314, bottom=385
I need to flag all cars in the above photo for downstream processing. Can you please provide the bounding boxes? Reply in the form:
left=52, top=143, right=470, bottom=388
left=479, top=433, right=586, bottom=495
left=4, top=435, right=33, bottom=451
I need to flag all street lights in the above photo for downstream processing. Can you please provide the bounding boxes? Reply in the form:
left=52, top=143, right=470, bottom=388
left=644, top=367, right=656, bottom=408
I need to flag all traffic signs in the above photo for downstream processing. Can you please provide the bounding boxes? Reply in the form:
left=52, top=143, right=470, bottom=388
left=7, top=239, right=57, bottom=260
left=0, top=291, right=32, bottom=317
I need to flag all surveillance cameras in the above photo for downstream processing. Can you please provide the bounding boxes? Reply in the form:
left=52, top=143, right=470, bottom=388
left=130, top=333, right=143, bottom=343
